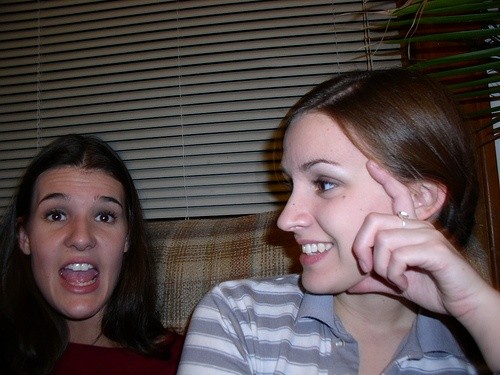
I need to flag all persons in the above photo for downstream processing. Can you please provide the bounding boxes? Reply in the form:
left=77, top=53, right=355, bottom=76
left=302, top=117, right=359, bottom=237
left=0, top=134, right=183, bottom=375
left=176, top=70, right=500, bottom=375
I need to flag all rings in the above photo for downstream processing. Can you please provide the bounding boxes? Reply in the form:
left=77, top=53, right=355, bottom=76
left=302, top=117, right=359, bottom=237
left=396, top=212, right=409, bottom=226
